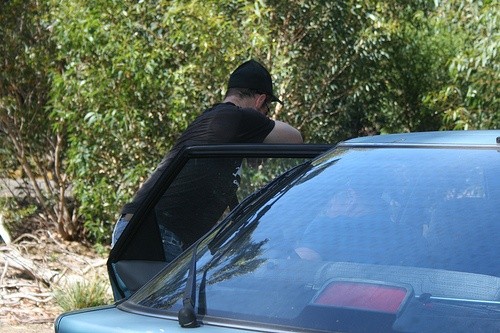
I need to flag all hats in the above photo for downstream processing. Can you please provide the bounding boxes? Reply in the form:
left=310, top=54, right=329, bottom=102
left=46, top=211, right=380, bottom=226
left=227, top=59, right=285, bottom=105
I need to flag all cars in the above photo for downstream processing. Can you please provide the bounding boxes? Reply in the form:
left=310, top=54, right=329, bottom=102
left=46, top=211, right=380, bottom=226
left=53, top=130, right=500, bottom=333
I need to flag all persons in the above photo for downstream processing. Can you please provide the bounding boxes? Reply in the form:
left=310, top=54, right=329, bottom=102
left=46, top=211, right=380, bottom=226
left=111, top=58, right=304, bottom=299
left=287, top=172, right=402, bottom=264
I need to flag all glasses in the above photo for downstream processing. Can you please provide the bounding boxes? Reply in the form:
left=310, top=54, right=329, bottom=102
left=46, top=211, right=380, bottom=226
left=264, top=101, right=274, bottom=114
